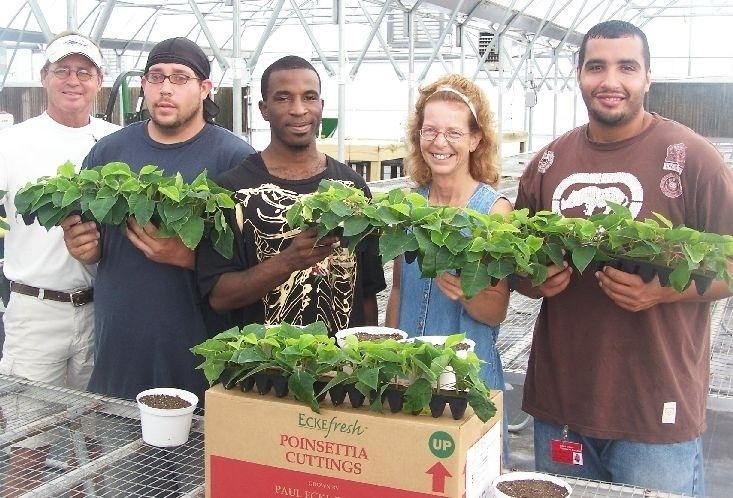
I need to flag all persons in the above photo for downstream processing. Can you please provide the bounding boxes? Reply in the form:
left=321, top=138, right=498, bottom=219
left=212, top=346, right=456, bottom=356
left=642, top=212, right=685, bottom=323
left=60, top=33, right=263, bottom=498
left=503, top=18, right=733, bottom=497
left=0, top=28, right=125, bottom=498
left=187, top=51, right=385, bottom=335
left=381, top=70, right=519, bottom=478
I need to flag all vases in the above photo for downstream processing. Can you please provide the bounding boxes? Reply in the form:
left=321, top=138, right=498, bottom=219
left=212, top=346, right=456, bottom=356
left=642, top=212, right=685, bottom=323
left=488, top=471, right=570, bottom=498
left=335, top=325, right=476, bottom=391
left=136, top=387, right=199, bottom=448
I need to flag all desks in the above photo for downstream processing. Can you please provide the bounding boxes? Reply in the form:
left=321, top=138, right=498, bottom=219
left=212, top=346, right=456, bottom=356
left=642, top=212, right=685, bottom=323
left=302, top=131, right=524, bottom=183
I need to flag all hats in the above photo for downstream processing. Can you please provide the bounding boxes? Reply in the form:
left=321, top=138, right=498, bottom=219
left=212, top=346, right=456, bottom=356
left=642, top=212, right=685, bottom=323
left=39, top=35, right=104, bottom=71
left=145, top=37, right=210, bottom=80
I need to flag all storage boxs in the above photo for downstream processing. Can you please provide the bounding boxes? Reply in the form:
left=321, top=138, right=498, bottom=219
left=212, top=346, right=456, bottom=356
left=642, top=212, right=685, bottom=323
left=203, top=381, right=504, bottom=498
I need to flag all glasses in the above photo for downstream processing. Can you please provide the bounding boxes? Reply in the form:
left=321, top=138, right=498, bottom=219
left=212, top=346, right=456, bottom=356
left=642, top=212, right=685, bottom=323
left=418, top=126, right=473, bottom=143
left=144, top=73, right=206, bottom=85
left=51, top=66, right=99, bottom=81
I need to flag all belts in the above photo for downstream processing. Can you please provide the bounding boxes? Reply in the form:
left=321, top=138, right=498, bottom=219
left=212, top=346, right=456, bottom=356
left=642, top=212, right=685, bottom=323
left=10, top=281, right=95, bottom=307
